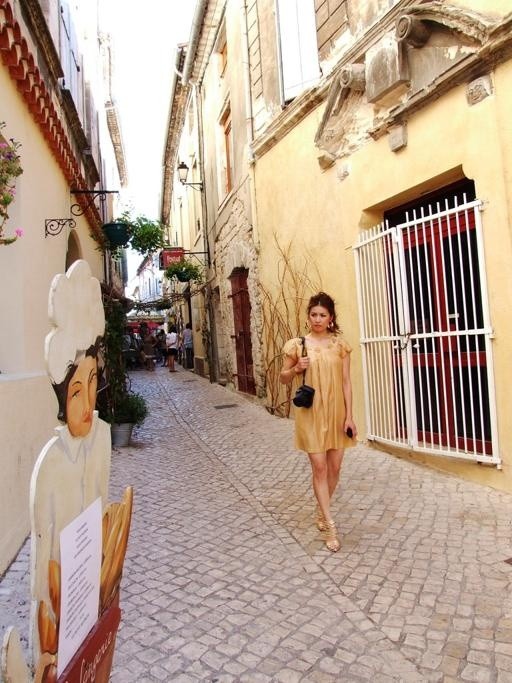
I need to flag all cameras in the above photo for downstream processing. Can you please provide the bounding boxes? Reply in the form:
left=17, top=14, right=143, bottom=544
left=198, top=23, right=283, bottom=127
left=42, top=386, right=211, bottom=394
left=293, top=385, right=315, bottom=408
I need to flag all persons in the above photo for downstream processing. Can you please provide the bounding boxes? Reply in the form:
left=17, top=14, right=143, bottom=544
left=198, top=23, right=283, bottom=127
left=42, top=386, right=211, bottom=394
left=278, top=294, right=357, bottom=551
left=144, top=323, right=193, bottom=372
left=30, top=346, right=112, bottom=661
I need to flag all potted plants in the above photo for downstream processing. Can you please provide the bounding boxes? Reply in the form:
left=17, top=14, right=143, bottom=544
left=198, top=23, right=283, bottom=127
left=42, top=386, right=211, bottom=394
left=165, top=256, right=203, bottom=284
left=111, top=388, right=148, bottom=446
left=90, top=214, right=164, bottom=253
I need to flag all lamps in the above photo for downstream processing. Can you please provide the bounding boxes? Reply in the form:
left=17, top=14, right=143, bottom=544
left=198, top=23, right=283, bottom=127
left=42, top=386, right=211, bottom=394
left=177, top=162, right=203, bottom=191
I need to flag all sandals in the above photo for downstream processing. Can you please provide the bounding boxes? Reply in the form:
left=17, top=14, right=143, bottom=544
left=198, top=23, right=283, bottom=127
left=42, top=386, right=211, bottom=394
left=316, top=512, right=326, bottom=531
left=325, top=521, right=340, bottom=552
